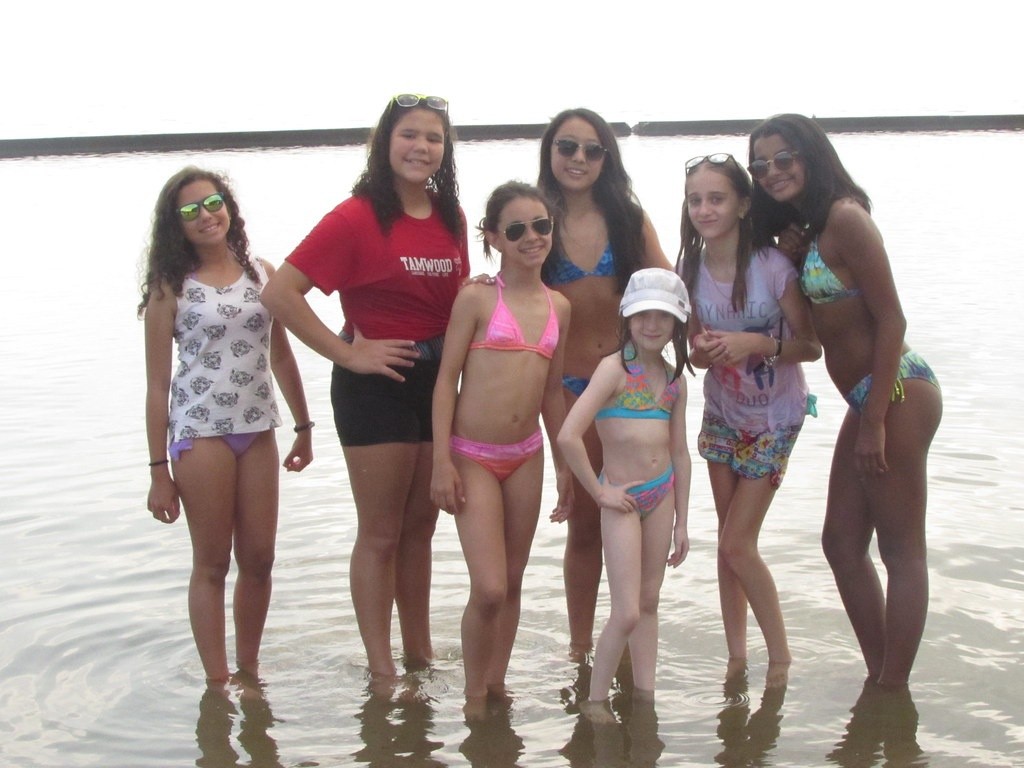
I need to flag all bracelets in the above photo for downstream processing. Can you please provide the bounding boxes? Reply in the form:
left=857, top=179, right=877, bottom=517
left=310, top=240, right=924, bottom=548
left=294, top=422, right=315, bottom=432
left=761, top=336, right=782, bottom=366
left=149, top=460, right=169, bottom=466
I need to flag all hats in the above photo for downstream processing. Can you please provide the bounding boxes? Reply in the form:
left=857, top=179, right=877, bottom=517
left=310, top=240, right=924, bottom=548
left=619, top=267, right=692, bottom=324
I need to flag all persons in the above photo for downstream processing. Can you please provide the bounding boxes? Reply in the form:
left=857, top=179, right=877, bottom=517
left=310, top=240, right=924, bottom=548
left=675, top=155, right=821, bottom=664
left=146, top=167, right=313, bottom=679
left=430, top=181, right=576, bottom=698
left=260, top=96, right=496, bottom=672
left=748, top=114, right=943, bottom=685
left=460, top=107, right=673, bottom=645
left=557, top=266, right=691, bottom=702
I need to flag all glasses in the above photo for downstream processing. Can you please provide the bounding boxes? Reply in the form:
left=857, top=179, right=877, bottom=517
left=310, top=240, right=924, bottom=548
left=496, top=218, right=554, bottom=242
left=389, top=93, right=449, bottom=118
left=747, top=149, right=800, bottom=179
left=685, top=153, right=747, bottom=187
left=552, top=139, right=609, bottom=162
left=175, top=191, right=224, bottom=221
